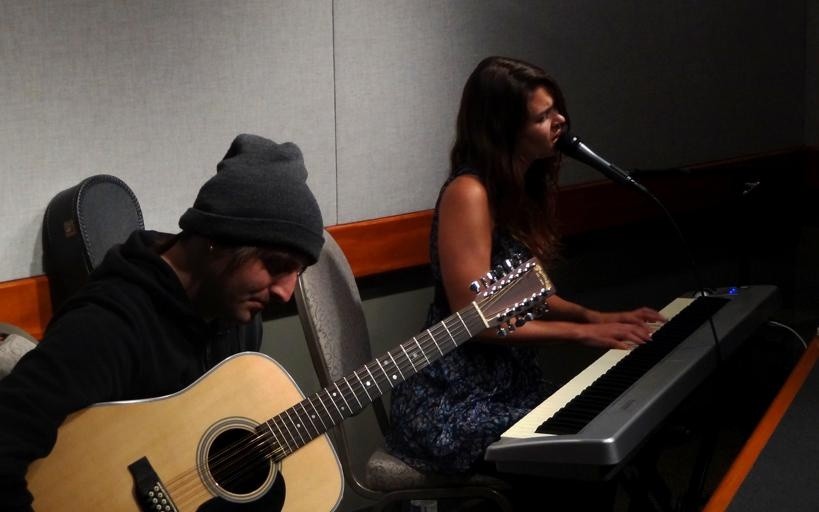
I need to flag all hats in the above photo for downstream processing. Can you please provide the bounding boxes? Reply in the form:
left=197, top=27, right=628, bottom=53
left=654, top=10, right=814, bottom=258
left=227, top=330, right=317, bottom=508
left=176, top=132, right=326, bottom=267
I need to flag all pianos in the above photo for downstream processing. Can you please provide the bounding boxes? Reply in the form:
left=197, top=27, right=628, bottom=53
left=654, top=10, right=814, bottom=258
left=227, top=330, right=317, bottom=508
left=483, top=285, right=776, bottom=484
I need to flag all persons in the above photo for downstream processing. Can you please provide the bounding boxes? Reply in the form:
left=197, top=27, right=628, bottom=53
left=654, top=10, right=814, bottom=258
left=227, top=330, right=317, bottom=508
left=5, top=131, right=327, bottom=511
left=386, top=56, right=669, bottom=478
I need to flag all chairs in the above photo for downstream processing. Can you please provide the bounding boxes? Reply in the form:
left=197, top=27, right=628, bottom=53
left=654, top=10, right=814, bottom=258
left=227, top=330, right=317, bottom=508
left=294, top=228, right=512, bottom=511
left=0, top=320, right=38, bottom=375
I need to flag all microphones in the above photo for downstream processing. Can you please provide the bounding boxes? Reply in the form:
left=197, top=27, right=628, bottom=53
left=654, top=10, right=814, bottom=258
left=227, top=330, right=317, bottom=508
left=554, top=131, right=632, bottom=187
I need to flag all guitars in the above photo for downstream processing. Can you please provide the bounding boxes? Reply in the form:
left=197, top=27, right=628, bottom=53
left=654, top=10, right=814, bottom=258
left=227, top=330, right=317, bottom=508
left=26, top=252, right=557, bottom=512
